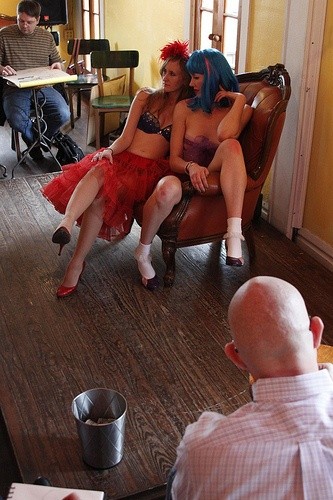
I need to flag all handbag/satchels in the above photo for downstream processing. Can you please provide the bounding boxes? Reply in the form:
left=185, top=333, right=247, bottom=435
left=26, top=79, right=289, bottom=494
left=66, top=59, right=89, bottom=74
left=53, top=132, right=85, bottom=171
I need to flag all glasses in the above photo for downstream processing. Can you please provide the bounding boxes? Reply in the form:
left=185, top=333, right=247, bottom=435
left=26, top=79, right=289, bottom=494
left=17, top=18, right=36, bottom=25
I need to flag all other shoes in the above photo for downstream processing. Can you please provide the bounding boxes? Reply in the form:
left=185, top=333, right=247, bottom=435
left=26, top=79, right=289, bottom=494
left=40, top=133, right=52, bottom=152
left=21, top=134, right=43, bottom=159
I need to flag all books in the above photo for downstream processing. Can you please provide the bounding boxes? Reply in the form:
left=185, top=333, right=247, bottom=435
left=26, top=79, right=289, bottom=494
left=7, top=483, right=104, bottom=500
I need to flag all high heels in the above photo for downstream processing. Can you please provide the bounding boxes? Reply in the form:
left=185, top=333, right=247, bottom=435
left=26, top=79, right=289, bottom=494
left=51, top=226, right=71, bottom=257
left=55, top=260, right=85, bottom=298
left=134, top=256, right=160, bottom=291
left=225, top=238, right=244, bottom=266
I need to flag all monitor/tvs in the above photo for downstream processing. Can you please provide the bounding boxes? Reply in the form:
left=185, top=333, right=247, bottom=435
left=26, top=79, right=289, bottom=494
left=36, top=0, right=68, bottom=26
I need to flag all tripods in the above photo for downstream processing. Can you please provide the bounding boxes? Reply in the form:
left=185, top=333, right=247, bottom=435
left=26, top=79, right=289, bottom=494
left=7, top=70, right=63, bottom=179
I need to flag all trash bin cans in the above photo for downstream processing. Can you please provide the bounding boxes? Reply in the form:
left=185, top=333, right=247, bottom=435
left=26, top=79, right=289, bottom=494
left=71, top=389, right=128, bottom=470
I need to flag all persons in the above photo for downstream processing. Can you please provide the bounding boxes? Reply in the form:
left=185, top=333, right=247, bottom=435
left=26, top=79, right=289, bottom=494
left=134, top=48, right=253, bottom=290
left=0, top=476, right=79, bottom=500
left=0, top=0, right=71, bottom=160
left=165, top=276, right=333, bottom=500
left=39, top=39, right=196, bottom=297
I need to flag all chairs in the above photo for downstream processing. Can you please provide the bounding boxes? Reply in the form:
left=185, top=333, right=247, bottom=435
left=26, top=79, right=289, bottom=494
left=128, top=63, right=291, bottom=284
left=67, top=39, right=110, bottom=129
left=91, top=50, right=139, bottom=151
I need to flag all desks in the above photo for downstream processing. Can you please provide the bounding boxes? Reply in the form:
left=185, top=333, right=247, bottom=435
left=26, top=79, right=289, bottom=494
left=6, top=81, right=63, bottom=181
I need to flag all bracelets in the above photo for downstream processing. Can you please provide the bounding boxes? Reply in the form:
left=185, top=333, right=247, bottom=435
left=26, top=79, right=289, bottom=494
left=104, top=148, right=113, bottom=156
left=184, top=161, right=195, bottom=175
